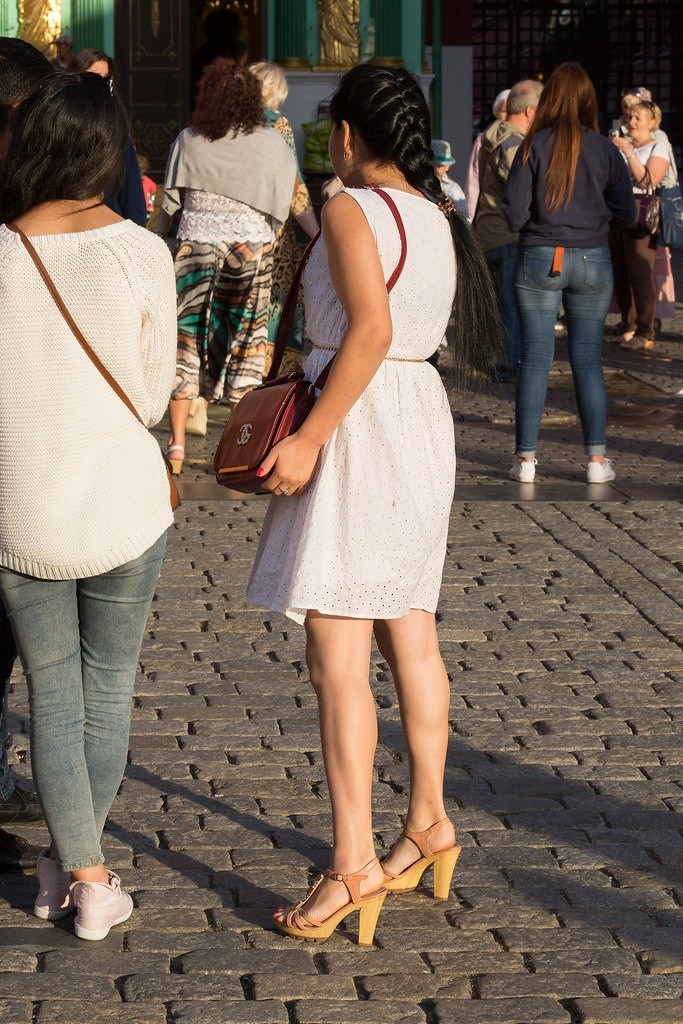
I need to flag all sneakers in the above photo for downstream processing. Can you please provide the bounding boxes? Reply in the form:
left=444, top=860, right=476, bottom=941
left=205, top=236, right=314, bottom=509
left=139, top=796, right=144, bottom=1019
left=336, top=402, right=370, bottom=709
left=510, top=458, right=538, bottom=483
left=34, top=848, right=76, bottom=919
left=69, top=870, right=132, bottom=941
left=586, top=458, right=616, bottom=484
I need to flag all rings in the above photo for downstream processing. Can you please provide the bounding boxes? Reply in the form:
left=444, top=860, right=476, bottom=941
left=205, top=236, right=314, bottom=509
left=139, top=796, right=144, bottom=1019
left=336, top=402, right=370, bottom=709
left=278, top=485, right=288, bottom=494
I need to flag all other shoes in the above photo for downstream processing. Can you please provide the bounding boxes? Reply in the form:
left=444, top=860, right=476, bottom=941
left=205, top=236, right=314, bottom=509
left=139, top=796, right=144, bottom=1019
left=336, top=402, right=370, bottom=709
left=620, top=335, right=654, bottom=350
left=603, top=328, right=635, bottom=342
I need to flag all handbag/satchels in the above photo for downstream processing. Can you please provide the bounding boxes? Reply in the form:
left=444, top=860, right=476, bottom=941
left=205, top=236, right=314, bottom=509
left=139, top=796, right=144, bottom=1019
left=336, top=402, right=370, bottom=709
left=614, top=165, right=660, bottom=235
left=161, top=451, right=181, bottom=512
left=650, top=144, right=683, bottom=247
left=214, top=372, right=321, bottom=495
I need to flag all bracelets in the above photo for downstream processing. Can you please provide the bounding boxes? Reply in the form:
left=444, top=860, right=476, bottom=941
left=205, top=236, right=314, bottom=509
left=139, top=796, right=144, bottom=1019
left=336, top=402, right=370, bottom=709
left=627, top=153, right=636, bottom=160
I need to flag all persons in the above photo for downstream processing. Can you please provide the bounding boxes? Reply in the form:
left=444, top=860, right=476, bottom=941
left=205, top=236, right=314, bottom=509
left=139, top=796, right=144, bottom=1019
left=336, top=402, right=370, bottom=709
left=501, top=62, right=637, bottom=483
left=0, top=69, right=177, bottom=940
left=430, top=138, right=467, bottom=216
left=67, top=48, right=145, bottom=228
left=161, top=58, right=297, bottom=476
left=0, top=37, right=59, bottom=819
left=245, top=64, right=460, bottom=947
left=49, top=36, right=73, bottom=70
left=465, top=80, right=545, bottom=381
left=185, top=62, right=320, bottom=436
left=608, top=87, right=678, bottom=349
left=138, top=156, right=157, bottom=219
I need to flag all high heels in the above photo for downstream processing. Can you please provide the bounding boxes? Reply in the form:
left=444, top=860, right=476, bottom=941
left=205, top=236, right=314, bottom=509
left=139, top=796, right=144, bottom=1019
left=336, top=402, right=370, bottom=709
left=380, top=820, right=461, bottom=902
left=185, top=397, right=208, bottom=436
left=274, top=858, right=388, bottom=946
left=164, top=446, right=185, bottom=475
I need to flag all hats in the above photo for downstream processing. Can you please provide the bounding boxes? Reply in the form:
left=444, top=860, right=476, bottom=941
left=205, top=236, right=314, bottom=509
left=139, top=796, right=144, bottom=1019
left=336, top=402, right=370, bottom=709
left=431, top=139, right=456, bottom=166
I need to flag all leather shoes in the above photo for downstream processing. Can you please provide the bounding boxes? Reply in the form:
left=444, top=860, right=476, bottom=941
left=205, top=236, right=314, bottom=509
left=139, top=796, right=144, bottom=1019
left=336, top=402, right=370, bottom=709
left=0, top=785, right=44, bottom=821
left=0, top=829, right=49, bottom=868
